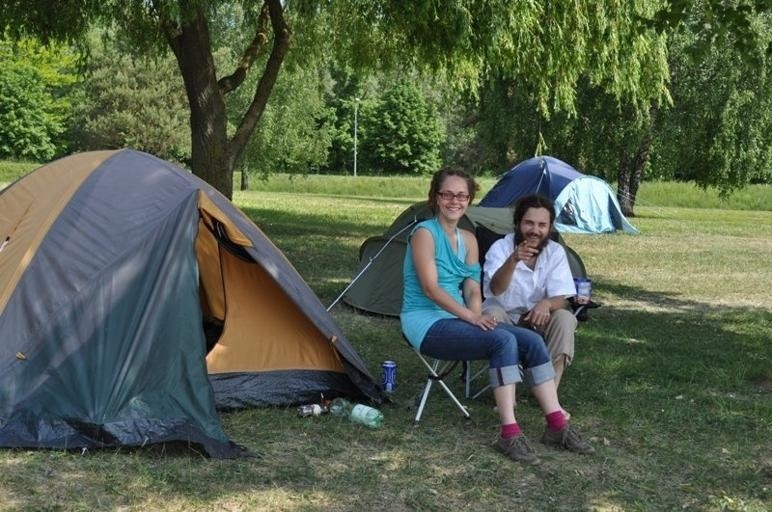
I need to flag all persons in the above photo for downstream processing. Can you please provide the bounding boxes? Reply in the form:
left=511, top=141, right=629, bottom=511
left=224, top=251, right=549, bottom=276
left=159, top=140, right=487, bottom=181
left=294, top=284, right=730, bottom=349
left=481, top=192, right=579, bottom=422
left=399, top=168, right=595, bottom=465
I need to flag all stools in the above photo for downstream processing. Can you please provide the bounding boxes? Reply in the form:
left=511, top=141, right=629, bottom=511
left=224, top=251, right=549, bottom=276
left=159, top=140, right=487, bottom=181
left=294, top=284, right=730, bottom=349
left=400, top=333, right=471, bottom=424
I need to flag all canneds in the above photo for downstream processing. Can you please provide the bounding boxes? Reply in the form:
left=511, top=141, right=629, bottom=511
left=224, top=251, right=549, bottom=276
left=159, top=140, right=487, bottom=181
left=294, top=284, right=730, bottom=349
left=382, top=361, right=397, bottom=392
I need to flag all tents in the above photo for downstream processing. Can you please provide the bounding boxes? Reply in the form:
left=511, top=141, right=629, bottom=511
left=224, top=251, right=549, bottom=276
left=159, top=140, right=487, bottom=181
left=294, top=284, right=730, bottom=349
left=344, top=198, right=588, bottom=319
left=1, top=146, right=394, bottom=460
left=478, top=154, right=642, bottom=238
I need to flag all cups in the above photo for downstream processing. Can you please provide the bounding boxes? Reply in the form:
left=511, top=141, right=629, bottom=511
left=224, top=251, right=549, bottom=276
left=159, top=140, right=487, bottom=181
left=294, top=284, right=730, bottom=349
left=574, top=278, right=591, bottom=303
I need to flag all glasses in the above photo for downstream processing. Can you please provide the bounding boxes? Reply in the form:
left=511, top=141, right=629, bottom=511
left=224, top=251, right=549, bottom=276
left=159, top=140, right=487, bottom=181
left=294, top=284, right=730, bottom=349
left=435, top=189, right=473, bottom=203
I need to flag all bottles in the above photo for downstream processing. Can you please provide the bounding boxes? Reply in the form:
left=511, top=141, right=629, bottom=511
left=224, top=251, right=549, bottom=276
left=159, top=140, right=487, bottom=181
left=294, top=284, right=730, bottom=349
left=322, top=397, right=384, bottom=427
left=299, top=403, right=330, bottom=416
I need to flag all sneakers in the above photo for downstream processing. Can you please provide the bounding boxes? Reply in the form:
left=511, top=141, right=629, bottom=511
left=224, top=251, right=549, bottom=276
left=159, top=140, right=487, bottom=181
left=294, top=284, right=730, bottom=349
left=490, top=434, right=541, bottom=466
left=493, top=397, right=572, bottom=421
left=540, top=427, right=598, bottom=456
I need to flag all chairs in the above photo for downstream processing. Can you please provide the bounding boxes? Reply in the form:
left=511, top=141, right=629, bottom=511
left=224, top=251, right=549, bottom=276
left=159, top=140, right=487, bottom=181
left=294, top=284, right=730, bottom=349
left=467, top=227, right=561, bottom=401
